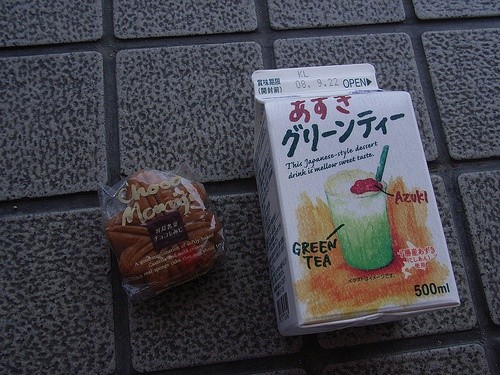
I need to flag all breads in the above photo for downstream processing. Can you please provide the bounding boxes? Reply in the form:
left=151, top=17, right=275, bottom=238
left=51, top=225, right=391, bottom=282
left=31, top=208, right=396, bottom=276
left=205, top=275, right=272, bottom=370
left=106, top=172, right=222, bottom=287
